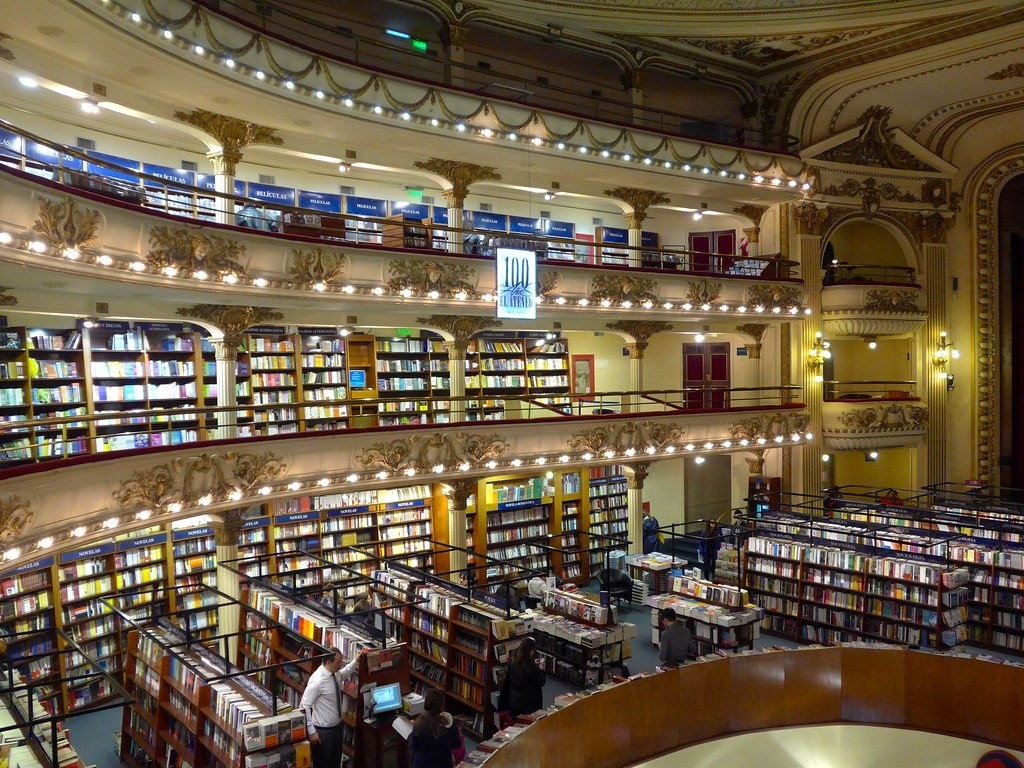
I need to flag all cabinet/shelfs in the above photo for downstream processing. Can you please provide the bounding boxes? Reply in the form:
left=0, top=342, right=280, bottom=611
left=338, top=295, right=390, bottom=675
left=374, top=336, right=430, bottom=427
left=647, top=518, right=766, bottom=658
left=217, top=550, right=432, bottom=768
left=821, top=484, right=1024, bottom=544
left=478, top=338, right=528, bottom=420
left=349, top=535, right=550, bottom=745
left=524, top=337, right=573, bottom=418
left=0, top=616, right=136, bottom=768
left=98, top=584, right=336, bottom=768
left=743, top=490, right=1024, bottom=658
left=0, top=485, right=434, bottom=719
left=236, top=333, right=348, bottom=437
left=0, top=316, right=236, bottom=469
left=345, top=334, right=380, bottom=429
left=923, top=481, right=1024, bottom=523
left=380, top=213, right=434, bottom=249
left=429, top=337, right=484, bottom=423
left=715, top=511, right=969, bottom=650
left=518, top=529, right=637, bottom=689
left=467, top=465, right=628, bottom=591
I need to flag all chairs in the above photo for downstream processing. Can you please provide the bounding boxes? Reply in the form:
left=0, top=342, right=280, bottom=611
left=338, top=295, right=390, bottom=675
left=592, top=408, right=619, bottom=416
left=597, top=569, right=634, bottom=609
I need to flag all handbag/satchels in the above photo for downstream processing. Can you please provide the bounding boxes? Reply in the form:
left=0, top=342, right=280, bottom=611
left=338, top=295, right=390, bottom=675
left=451, top=735, right=468, bottom=766
left=697, top=548, right=705, bottom=564
left=499, top=660, right=512, bottom=713
left=658, top=532, right=665, bottom=545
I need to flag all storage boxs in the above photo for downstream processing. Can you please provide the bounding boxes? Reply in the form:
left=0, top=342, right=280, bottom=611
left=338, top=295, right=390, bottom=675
left=725, top=271, right=729, bottom=274
left=730, top=260, right=769, bottom=276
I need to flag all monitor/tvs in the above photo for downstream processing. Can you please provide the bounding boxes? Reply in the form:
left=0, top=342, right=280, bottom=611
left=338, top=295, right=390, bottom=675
left=756, top=505, right=769, bottom=518
left=349, top=370, right=365, bottom=391
left=370, top=682, right=402, bottom=714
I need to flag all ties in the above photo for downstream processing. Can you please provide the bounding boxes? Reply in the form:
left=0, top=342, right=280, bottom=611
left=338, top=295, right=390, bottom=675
left=332, top=673, right=341, bottom=718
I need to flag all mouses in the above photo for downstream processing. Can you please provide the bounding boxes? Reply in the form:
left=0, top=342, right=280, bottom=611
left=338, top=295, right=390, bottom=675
left=367, top=388, right=372, bottom=390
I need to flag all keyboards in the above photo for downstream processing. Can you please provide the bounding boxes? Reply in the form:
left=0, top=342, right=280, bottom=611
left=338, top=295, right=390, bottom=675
left=373, top=716, right=398, bottom=727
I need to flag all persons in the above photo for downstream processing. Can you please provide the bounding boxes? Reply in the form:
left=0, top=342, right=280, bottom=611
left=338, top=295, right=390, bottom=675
left=299, top=646, right=363, bottom=768
left=698, top=518, right=725, bottom=580
left=881, top=490, right=904, bottom=506
left=730, top=509, right=748, bottom=546
left=496, top=571, right=519, bottom=610
left=409, top=689, right=467, bottom=767
left=459, top=570, right=474, bottom=587
left=321, top=583, right=346, bottom=614
left=353, top=598, right=372, bottom=624
left=823, top=486, right=844, bottom=517
left=658, top=607, right=697, bottom=664
left=506, top=636, right=546, bottom=722
left=642, top=511, right=659, bottom=555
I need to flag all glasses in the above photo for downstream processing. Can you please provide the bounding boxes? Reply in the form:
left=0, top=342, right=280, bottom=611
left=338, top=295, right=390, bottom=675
left=710, top=521, right=716, bottom=524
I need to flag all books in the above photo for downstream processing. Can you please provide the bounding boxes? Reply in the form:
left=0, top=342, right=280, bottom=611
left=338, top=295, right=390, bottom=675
left=0, top=160, right=770, bottom=276
left=0, top=330, right=568, bottom=464
left=0, top=466, right=1024, bottom=768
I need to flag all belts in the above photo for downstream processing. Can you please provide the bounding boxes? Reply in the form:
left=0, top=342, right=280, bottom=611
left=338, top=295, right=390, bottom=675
left=315, top=722, right=342, bottom=730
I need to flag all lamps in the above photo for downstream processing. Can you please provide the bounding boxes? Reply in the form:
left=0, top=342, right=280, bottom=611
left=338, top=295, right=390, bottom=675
left=946, top=373, right=955, bottom=392
left=695, top=213, right=703, bottom=221
left=341, top=327, right=355, bottom=336
left=695, top=332, right=705, bottom=341
left=546, top=333, right=558, bottom=339
left=696, top=457, right=705, bottom=465
left=546, top=471, right=558, bottom=478
left=807, top=332, right=831, bottom=382
left=831, top=255, right=840, bottom=266
left=822, top=454, right=833, bottom=462
left=338, top=163, right=352, bottom=174
left=932, top=331, right=960, bottom=379
left=83, top=319, right=100, bottom=328
left=544, top=193, right=557, bottom=200
left=867, top=337, right=879, bottom=350
left=864, top=451, right=878, bottom=463
